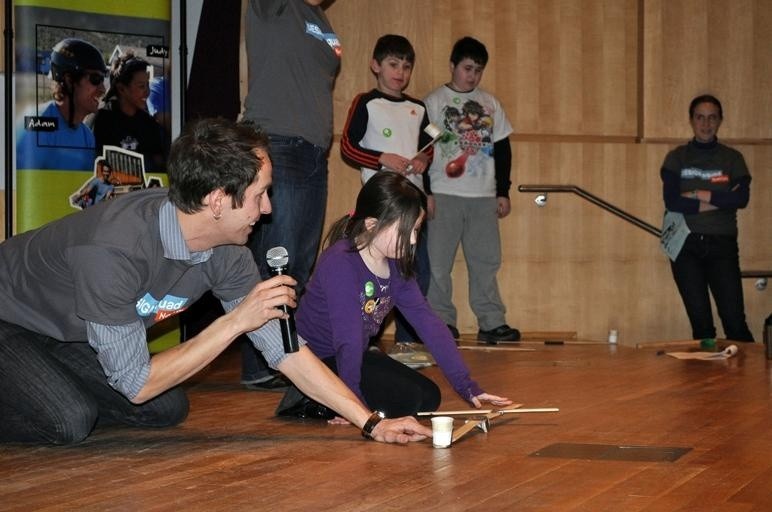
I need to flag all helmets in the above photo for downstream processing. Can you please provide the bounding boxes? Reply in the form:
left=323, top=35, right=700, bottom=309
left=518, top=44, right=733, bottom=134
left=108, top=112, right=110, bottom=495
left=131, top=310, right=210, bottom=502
left=51, top=38, right=106, bottom=81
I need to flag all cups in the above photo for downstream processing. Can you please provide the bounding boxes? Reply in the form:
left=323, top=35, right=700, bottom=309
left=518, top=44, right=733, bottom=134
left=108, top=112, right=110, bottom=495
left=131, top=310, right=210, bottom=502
left=432, top=416, right=455, bottom=448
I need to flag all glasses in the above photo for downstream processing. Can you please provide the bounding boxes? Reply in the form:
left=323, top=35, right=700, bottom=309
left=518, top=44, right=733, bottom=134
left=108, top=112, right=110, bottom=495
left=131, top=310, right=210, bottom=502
left=85, top=72, right=104, bottom=86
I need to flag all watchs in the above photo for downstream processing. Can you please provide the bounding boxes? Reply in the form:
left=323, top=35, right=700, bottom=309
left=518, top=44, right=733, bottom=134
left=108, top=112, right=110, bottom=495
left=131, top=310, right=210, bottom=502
left=361, top=410, right=385, bottom=441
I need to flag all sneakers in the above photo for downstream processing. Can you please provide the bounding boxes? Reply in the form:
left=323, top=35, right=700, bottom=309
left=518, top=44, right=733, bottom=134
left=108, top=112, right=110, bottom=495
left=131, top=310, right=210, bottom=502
left=246, top=375, right=327, bottom=419
left=447, top=324, right=460, bottom=339
left=475, top=324, right=520, bottom=344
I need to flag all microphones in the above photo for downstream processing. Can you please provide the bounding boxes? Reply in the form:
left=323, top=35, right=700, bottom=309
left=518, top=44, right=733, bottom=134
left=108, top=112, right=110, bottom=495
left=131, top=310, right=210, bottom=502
left=266, top=246, right=300, bottom=353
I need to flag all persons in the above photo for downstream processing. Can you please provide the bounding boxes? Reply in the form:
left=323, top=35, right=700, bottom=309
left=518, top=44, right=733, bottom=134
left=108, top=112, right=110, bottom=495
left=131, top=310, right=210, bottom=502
left=426, top=36, right=521, bottom=345
left=149, top=58, right=171, bottom=132
left=0, top=117, right=433, bottom=448
left=275, top=170, right=513, bottom=426
left=93, top=54, right=169, bottom=173
left=82, top=164, right=114, bottom=208
left=660, top=94, right=754, bottom=342
left=17, top=38, right=110, bottom=171
left=339, top=34, right=433, bottom=345
left=239, top=0, right=342, bottom=393
